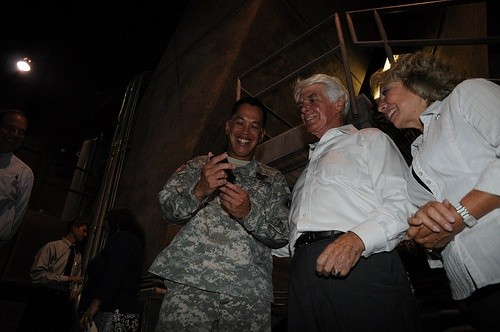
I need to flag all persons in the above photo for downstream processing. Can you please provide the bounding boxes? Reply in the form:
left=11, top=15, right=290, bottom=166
left=147, top=96, right=292, bottom=332
left=0, top=109, right=35, bottom=270
left=76, top=207, right=146, bottom=332
left=271, top=74, right=424, bottom=332
left=20, top=217, right=87, bottom=332
left=368, top=51, right=500, bottom=332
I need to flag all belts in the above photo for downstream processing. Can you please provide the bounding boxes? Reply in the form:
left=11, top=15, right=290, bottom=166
left=296, top=230, right=345, bottom=246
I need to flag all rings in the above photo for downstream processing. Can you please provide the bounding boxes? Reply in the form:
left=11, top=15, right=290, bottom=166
left=427, top=248, right=433, bottom=254
left=332, top=269, right=338, bottom=276
left=85, top=317, right=88, bottom=319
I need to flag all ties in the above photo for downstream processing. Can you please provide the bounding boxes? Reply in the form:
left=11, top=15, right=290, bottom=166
left=64, top=243, right=75, bottom=276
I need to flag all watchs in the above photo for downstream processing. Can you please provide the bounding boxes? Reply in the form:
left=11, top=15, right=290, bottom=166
left=449, top=198, right=477, bottom=228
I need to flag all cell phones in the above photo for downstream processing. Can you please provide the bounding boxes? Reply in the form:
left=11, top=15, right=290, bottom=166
left=209, top=154, right=236, bottom=184
